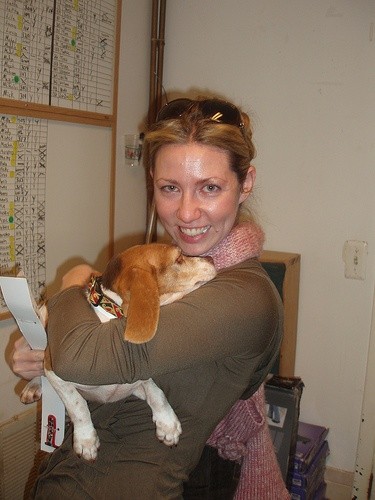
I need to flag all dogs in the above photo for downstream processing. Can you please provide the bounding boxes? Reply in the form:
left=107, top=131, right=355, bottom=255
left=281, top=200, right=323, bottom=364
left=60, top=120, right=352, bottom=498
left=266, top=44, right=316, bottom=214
left=19, top=243, right=217, bottom=461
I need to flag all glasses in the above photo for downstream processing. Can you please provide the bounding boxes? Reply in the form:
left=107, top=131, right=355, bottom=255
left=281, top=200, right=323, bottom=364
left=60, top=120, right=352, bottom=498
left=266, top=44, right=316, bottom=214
left=154, top=98, right=253, bottom=156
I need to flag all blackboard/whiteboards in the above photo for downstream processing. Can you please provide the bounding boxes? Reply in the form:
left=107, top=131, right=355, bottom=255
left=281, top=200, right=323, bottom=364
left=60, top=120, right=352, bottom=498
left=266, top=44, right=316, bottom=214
left=0, top=0, right=122, bottom=321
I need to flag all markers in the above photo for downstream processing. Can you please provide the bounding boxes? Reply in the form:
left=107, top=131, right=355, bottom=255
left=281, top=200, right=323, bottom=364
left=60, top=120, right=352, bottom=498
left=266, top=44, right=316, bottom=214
left=137, top=133, right=144, bottom=161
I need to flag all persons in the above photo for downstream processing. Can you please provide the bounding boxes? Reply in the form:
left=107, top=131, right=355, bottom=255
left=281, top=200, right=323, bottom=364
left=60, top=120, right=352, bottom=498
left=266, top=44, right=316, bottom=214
left=12, top=98, right=284, bottom=500
